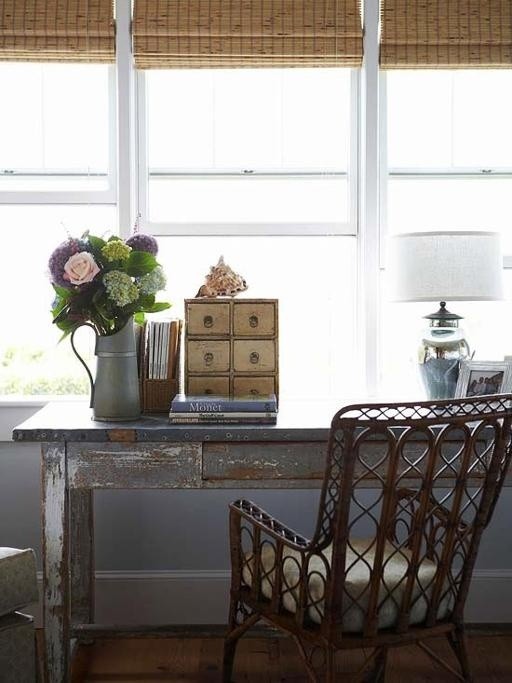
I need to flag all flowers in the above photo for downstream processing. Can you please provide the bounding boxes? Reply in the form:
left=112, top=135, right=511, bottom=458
left=48, top=230, right=171, bottom=345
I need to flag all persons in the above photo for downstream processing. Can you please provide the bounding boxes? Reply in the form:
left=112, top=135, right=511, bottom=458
left=467, top=376, right=496, bottom=395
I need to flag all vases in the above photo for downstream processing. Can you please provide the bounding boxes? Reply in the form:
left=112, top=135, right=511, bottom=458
left=71, top=315, right=141, bottom=422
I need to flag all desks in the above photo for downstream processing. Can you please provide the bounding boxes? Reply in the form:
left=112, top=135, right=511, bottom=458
left=11, top=394, right=512, bottom=683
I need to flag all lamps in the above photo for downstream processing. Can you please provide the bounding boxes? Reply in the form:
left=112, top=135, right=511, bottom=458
left=382, top=229, right=501, bottom=405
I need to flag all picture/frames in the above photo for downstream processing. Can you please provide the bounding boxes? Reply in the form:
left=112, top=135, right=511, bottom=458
left=454, top=355, right=512, bottom=399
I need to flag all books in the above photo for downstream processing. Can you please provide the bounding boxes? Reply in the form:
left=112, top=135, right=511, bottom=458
left=169, top=417, right=278, bottom=424
left=171, top=393, right=277, bottom=412
left=132, top=317, right=181, bottom=381
left=168, top=407, right=278, bottom=417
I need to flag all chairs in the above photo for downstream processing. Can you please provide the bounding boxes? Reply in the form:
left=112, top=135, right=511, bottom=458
left=220, top=394, right=512, bottom=683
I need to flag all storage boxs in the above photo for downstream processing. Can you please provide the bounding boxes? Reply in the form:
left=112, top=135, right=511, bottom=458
left=184, top=294, right=280, bottom=400
